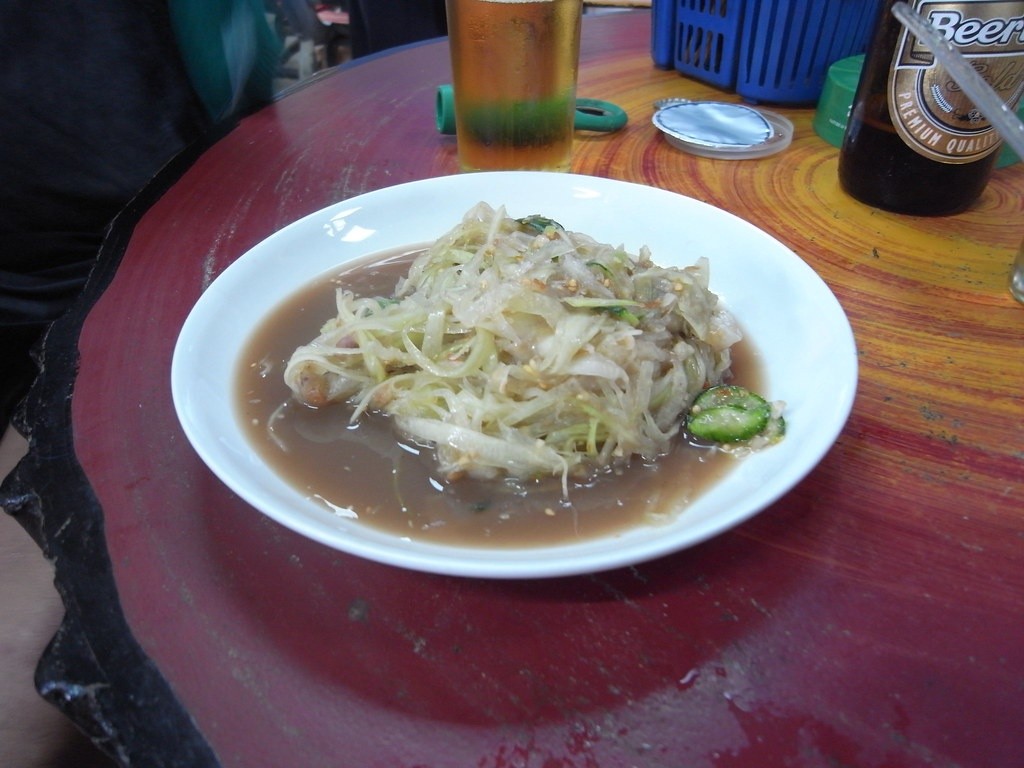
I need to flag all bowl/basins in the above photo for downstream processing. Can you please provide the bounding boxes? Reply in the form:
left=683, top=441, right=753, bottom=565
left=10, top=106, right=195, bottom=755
left=168, top=172, right=859, bottom=580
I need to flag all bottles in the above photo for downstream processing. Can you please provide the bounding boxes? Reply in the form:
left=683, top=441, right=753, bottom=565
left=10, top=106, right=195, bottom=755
left=837, top=0, right=1024, bottom=217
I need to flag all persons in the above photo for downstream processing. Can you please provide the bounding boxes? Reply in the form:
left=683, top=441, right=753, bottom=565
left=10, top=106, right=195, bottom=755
left=1, top=0, right=281, bottom=768
left=346, top=0, right=447, bottom=57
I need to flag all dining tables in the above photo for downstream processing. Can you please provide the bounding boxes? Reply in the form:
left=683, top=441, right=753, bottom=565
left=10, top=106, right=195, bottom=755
left=0, top=0, right=1024, bottom=768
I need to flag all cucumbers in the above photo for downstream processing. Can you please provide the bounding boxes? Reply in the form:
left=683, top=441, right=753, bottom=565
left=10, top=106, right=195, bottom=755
left=686, top=384, right=776, bottom=443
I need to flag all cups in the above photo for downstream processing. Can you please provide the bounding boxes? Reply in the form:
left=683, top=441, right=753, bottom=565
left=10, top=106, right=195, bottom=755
left=445, top=0, right=584, bottom=172
